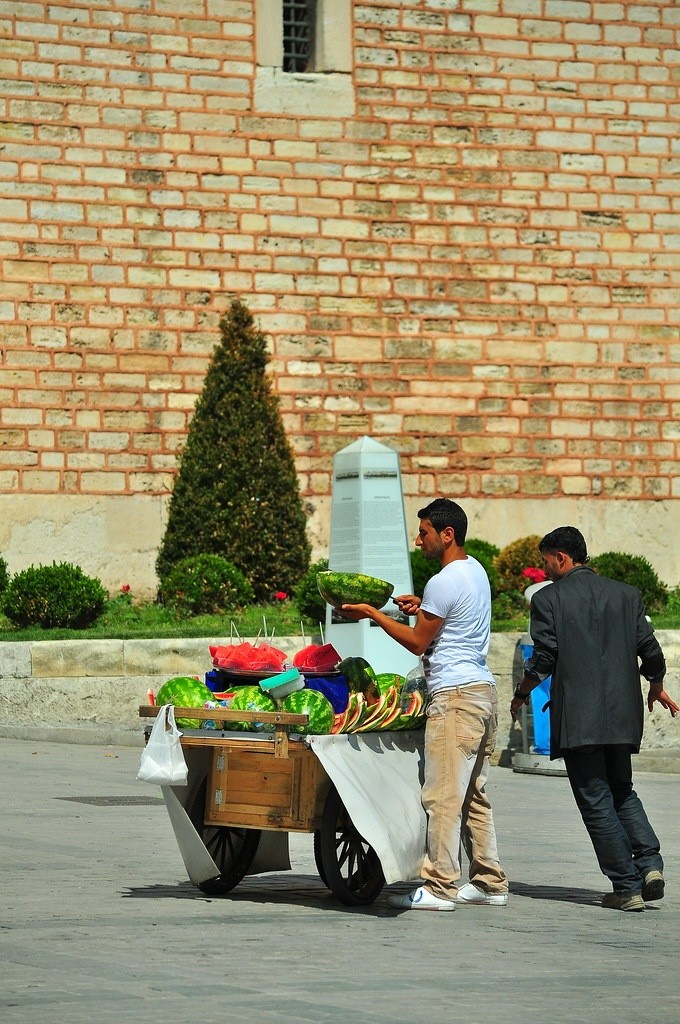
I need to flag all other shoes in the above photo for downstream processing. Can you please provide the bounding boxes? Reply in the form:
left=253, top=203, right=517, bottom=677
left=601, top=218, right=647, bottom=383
left=641, top=871, right=665, bottom=902
left=602, top=892, right=646, bottom=910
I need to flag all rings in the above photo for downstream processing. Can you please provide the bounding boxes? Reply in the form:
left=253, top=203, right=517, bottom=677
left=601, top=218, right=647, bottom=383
left=511, top=711, right=515, bottom=713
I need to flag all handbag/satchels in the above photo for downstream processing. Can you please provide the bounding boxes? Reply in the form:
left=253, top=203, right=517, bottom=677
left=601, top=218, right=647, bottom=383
left=136, top=704, right=189, bottom=786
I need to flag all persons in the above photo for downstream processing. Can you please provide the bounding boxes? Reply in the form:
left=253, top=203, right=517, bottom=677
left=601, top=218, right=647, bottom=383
left=511, top=526, right=680, bottom=912
left=331, top=497, right=510, bottom=912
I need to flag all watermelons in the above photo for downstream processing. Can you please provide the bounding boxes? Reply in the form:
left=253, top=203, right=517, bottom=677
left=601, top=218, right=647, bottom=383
left=155, top=571, right=407, bottom=736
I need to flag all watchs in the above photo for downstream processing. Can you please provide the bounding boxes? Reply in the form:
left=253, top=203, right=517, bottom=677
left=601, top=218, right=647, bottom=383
left=514, top=682, right=530, bottom=700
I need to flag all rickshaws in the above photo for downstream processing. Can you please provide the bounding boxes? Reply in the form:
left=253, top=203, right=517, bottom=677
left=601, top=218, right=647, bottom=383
left=137, top=704, right=425, bottom=907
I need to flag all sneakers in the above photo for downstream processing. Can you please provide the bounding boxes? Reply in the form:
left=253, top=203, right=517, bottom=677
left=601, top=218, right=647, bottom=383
left=385, top=887, right=455, bottom=910
left=455, top=883, right=508, bottom=906
